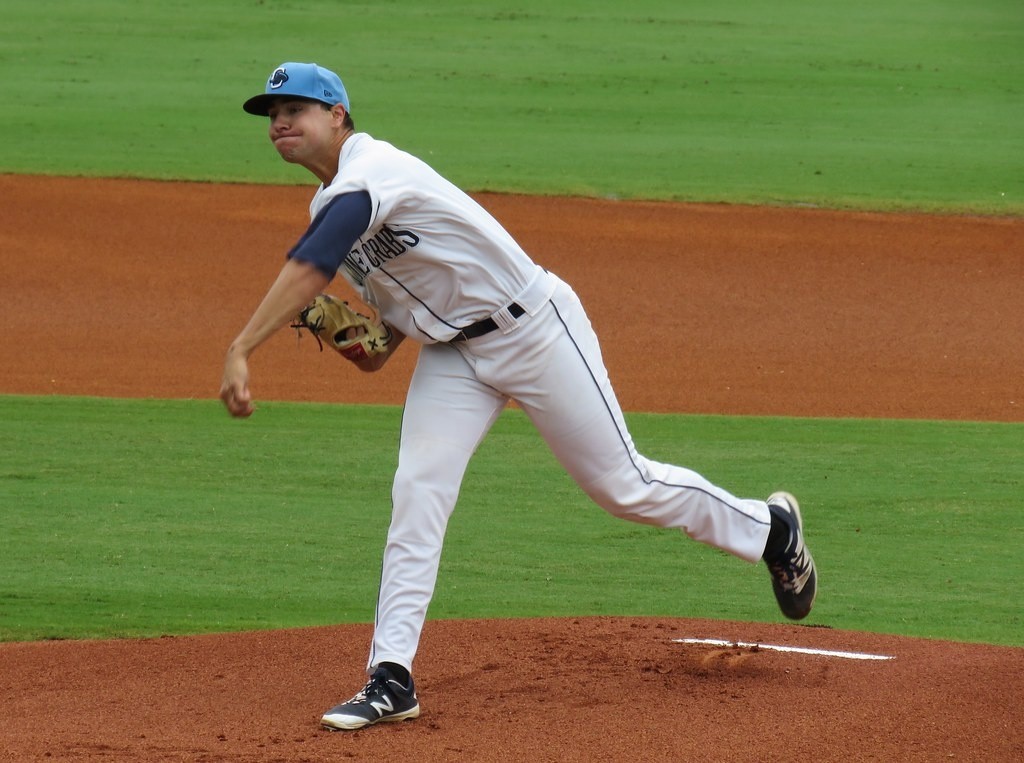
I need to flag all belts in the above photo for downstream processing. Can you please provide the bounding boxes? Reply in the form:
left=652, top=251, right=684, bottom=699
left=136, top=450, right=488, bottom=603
left=448, top=302, right=525, bottom=341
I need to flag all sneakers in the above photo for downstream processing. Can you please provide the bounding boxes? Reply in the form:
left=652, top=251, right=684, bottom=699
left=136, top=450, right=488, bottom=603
left=766, top=492, right=819, bottom=620
left=322, top=667, right=420, bottom=730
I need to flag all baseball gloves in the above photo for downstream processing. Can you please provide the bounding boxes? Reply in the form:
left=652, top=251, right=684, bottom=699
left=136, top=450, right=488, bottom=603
left=295, top=293, right=394, bottom=362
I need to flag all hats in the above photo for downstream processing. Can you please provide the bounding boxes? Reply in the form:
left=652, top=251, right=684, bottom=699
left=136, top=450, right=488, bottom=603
left=243, top=63, right=349, bottom=116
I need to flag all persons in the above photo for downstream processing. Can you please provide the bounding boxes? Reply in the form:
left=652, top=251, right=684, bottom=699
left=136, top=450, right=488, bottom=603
left=219, top=61, right=818, bottom=733
